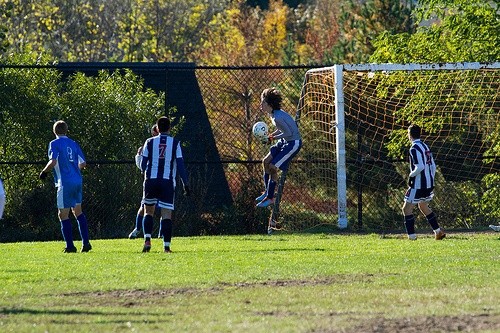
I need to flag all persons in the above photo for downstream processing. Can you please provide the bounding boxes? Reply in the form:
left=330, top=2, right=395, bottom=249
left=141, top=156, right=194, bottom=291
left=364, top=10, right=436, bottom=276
left=0, top=179, right=5, bottom=219
left=402, top=123, right=446, bottom=241
left=129, top=116, right=192, bottom=254
left=39, top=120, right=92, bottom=253
left=488, top=224, right=500, bottom=232
left=252, top=87, right=302, bottom=208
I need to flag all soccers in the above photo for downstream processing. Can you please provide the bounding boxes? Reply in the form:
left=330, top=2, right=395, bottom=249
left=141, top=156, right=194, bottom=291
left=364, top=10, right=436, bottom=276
left=253, top=122, right=269, bottom=138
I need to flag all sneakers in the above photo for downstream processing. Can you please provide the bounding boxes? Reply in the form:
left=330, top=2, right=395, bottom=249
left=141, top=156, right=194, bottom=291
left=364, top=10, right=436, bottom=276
left=62, top=247, right=77, bottom=254
left=158, top=235, right=163, bottom=239
left=128, top=228, right=141, bottom=239
left=256, top=196, right=274, bottom=207
left=255, top=193, right=267, bottom=201
left=142, top=241, right=151, bottom=253
left=435, top=230, right=446, bottom=240
left=164, top=250, right=172, bottom=253
left=409, top=237, right=417, bottom=240
left=80, top=242, right=92, bottom=253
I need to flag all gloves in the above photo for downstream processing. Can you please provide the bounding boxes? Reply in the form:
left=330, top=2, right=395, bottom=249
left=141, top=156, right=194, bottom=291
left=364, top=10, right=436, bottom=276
left=39, top=171, right=47, bottom=181
left=183, top=186, right=191, bottom=197
left=408, top=177, right=415, bottom=188
left=260, top=134, right=273, bottom=147
